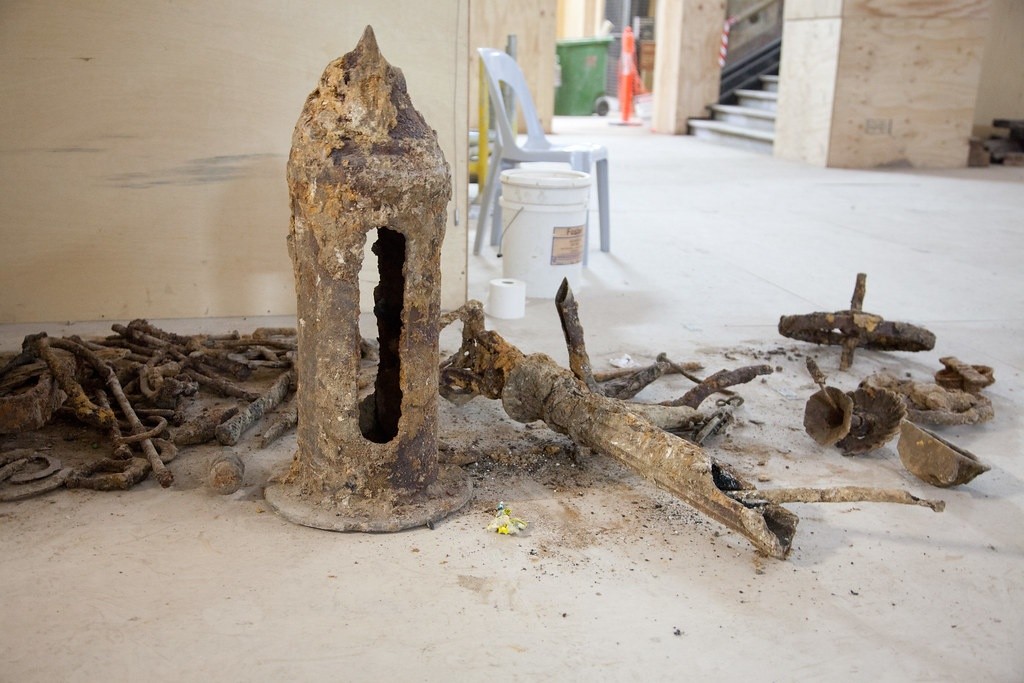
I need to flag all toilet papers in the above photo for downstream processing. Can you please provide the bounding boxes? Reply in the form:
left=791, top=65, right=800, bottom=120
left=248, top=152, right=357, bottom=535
left=484, top=277, right=526, bottom=319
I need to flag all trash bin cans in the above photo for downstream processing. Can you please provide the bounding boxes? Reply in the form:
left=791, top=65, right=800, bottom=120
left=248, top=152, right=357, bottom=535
left=553, top=36, right=616, bottom=115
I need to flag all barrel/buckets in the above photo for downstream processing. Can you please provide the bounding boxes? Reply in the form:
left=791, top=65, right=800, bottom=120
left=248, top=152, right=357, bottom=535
left=496, top=168, right=592, bottom=299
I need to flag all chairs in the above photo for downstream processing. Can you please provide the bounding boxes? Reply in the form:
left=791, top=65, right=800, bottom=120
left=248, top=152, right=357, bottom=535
left=474, top=47, right=611, bottom=257
